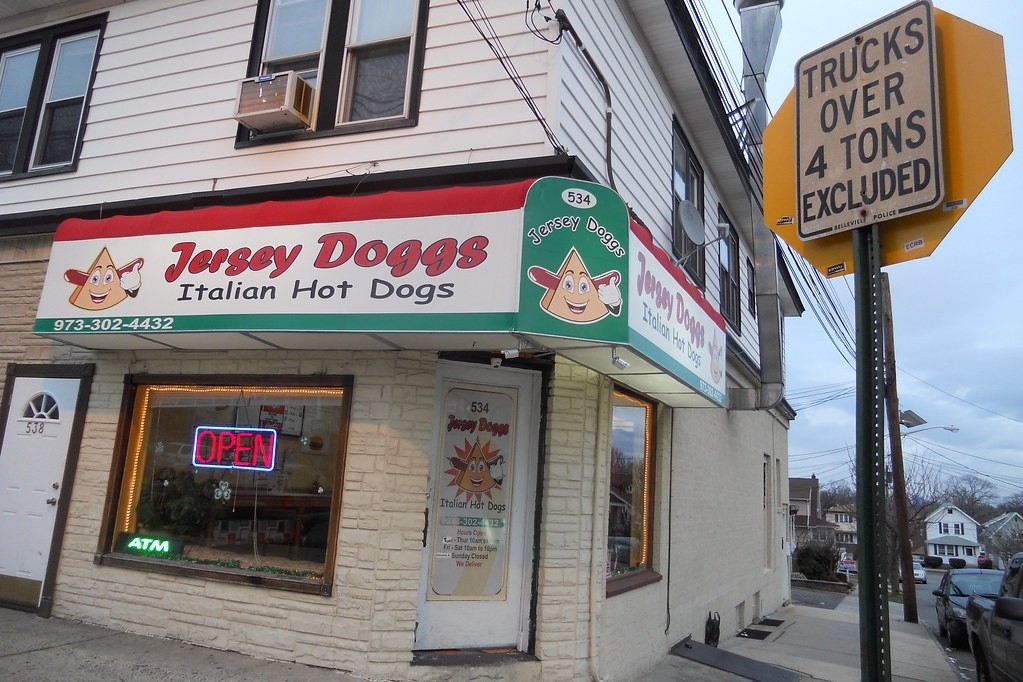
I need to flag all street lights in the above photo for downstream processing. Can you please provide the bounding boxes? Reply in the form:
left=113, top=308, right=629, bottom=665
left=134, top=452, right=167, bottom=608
left=894, top=424, right=960, bottom=624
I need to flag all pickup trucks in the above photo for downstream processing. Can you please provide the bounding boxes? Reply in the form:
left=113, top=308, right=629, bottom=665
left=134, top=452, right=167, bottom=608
left=966, top=551, right=1023, bottom=682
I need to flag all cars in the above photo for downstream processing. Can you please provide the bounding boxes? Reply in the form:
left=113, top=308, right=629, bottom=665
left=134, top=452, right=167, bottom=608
left=839, top=560, right=858, bottom=575
left=898, top=554, right=928, bottom=584
left=932, top=568, right=1006, bottom=650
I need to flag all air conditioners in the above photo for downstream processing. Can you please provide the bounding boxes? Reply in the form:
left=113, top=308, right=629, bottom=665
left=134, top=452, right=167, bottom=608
left=233, top=70, right=315, bottom=133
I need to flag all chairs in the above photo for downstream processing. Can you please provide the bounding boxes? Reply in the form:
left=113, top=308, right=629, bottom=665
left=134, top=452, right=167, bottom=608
left=990, top=581, right=1001, bottom=594
left=953, top=580, right=969, bottom=594
